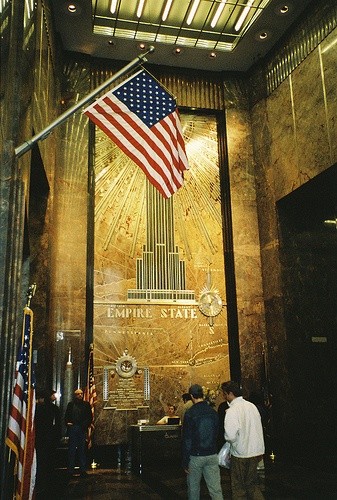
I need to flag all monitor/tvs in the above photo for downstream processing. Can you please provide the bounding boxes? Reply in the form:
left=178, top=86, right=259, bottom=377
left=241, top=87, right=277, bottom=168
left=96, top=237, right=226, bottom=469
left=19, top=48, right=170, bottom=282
left=168, top=418, right=180, bottom=425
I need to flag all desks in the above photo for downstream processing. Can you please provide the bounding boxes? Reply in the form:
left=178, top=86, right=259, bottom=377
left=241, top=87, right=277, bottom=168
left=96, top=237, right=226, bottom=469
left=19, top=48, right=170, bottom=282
left=127, top=426, right=182, bottom=482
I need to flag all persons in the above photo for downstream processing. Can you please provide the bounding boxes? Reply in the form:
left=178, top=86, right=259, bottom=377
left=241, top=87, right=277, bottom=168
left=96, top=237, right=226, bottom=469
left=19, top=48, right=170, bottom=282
left=64, top=389, right=92, bottom=479
left=179, top=384, right=223, bottom=500
left=35, top=388, right=62, bottom=478
left=221, top=380, right=265, bottom=500
left=217, top=398, right=230, bottom=435
left=177, top=393, right=194, bottom=419
left=155, top=402, right=181, bottom=425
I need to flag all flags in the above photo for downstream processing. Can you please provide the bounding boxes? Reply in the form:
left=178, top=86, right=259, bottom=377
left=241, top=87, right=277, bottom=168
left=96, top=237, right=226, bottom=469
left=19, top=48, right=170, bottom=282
left=81, top=69, right=190, bottom=200
left=82, top=351, right=100, bottom=450
left=4, top=305, right=42, bottom=500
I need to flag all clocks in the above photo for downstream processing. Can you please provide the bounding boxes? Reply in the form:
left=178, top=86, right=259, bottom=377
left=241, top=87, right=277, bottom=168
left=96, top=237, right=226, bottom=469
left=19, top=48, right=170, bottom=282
left=114, top=355, right=138, bottom=378
left=197, top=291, right=222, bottom=317
